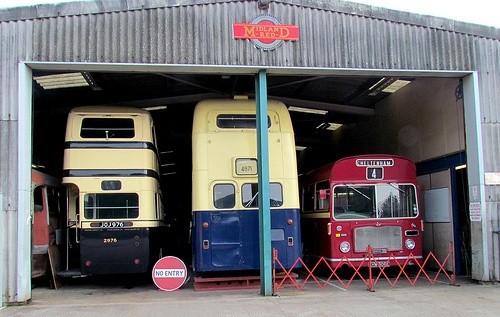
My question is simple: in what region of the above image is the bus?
[52,101,168,287]
[188,95,305,292]
[298,154,426,273]
